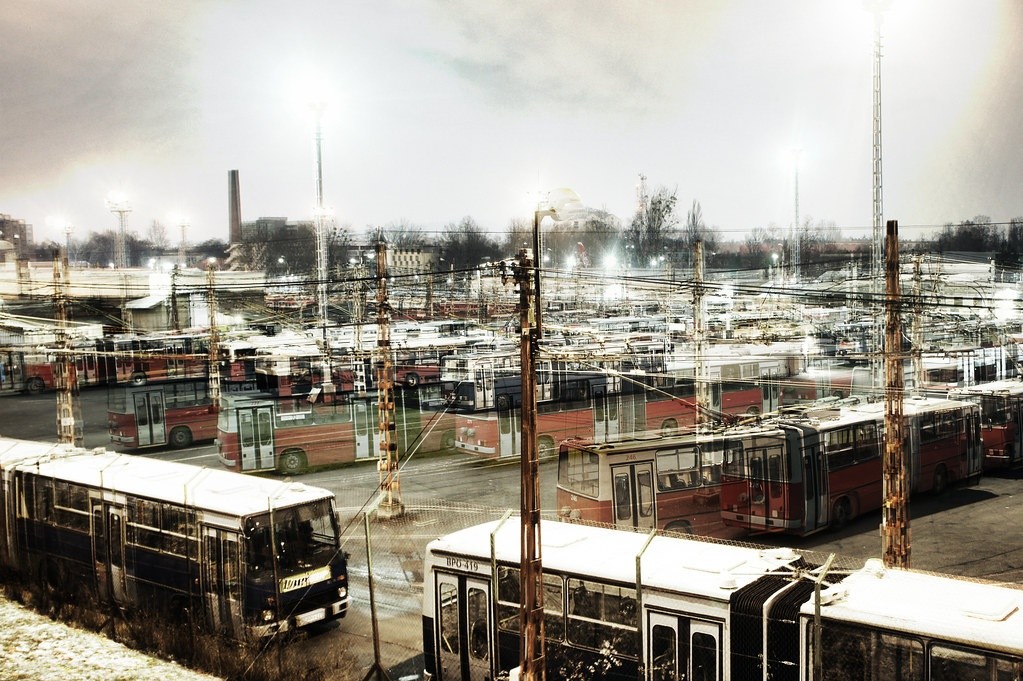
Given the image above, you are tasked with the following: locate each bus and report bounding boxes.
[0,273,1023,477]
[0,436,349,645]
[423,511,1023,681]
[558,397,983,533]
